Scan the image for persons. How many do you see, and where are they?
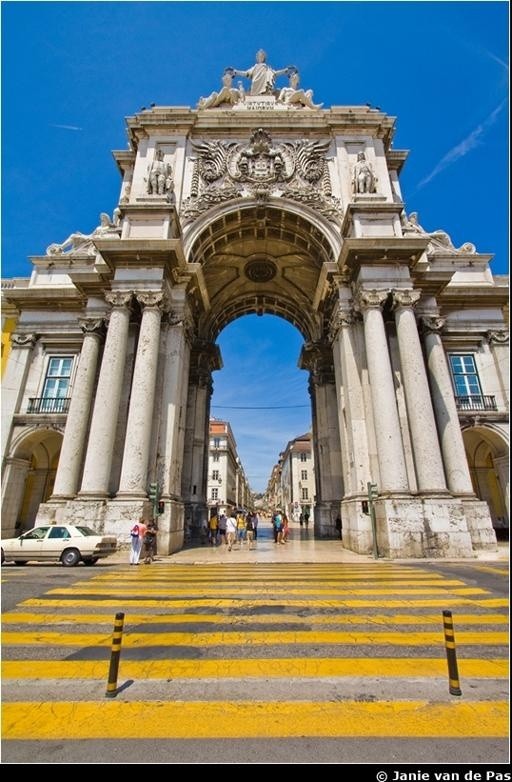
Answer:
[299,512,309,525]
[130,518,158,565]
[196,49,325,111]
[408,212,452,247]
[147,150,172,195]
[201,511,258,551]
[351,151,378,193]
[271,511,288,545]
[336,515,341,541]
[52,212,110,255]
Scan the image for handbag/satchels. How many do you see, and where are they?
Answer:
[131,525,139,536]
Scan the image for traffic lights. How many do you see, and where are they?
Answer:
[146,481,157,501]
[157,501,164,514]
[362,500,369,514]
[370,482,377,501]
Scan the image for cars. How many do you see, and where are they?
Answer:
[0,524,119,568]
